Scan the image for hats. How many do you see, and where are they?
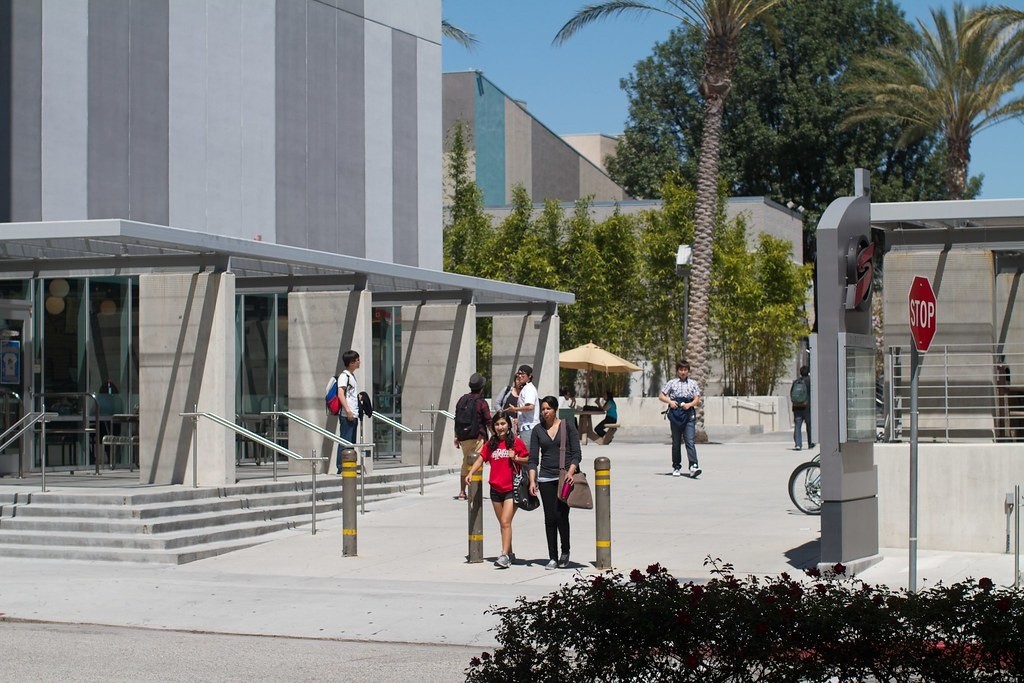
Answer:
[469,373,486,390]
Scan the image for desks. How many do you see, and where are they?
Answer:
[109,413,139,467]
[575,411,605,445]
[236,414,283,464]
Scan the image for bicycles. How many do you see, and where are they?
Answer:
[787,453,824,515]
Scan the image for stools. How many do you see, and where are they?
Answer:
[265,430,288,464]
[102,435,139,471]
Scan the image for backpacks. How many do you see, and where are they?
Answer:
[791,378,808,404]
[455,392,483,437]
[325,372,357,414]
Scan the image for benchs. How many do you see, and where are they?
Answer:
[604,424,620,445]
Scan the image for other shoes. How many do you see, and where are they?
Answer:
[795,446,801,450]
[808,443,816,449]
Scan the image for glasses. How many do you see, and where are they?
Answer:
[516,372,527,376]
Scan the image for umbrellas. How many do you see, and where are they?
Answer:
[559,340,644,405]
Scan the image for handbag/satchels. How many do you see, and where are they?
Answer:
[558,470,593,509]
[666,404,687,427]
[513,470,541,511]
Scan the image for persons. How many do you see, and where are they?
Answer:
[465,412,529,569]
[594,393,618,438]
[659,360,702,478]
[454,375,495,500]
[336,350,363,477]
[560,391,578,429]
[791,366,816,449]
[495,365,540,451]
[529,396,582,569]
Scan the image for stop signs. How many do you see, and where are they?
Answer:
[908,275,938,353]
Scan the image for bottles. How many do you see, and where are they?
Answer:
[561,478,573,501]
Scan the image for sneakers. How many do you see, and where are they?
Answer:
[689,463,702,479]
[494,555,511,567]
[498,554,517,562]
[544,559,558,570]
[559,551,570,568]
[673,468,680,476]
